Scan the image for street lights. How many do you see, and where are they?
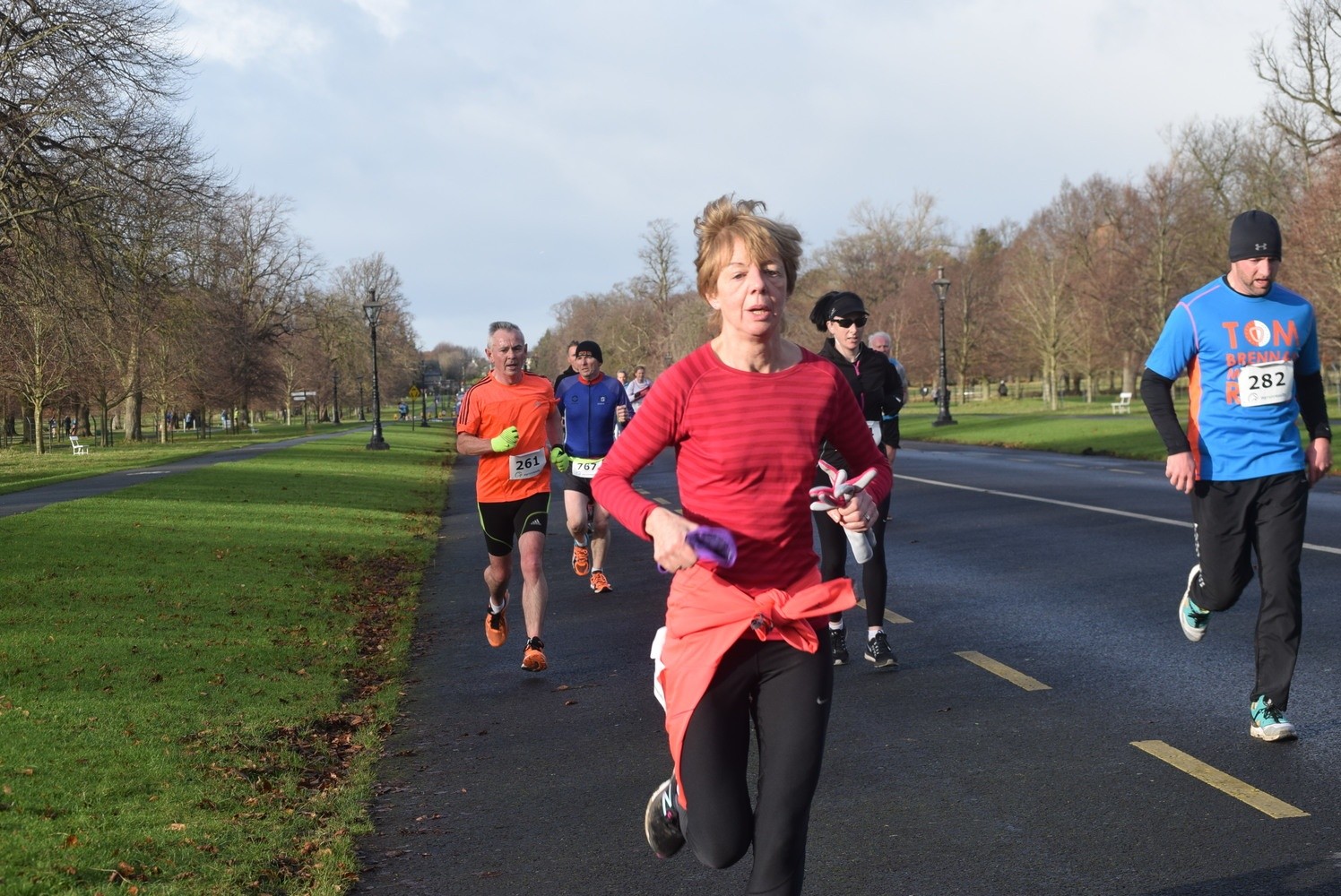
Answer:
[930,266,959,427]
[360,287,391,451]
[417,359,431,427]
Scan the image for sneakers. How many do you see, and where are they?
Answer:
[520,636,547,671]
[485,589,509,648]
[590,570,613,593]
[829,619,848,664]
[864,630,899,672]
[644,764,685,860]
[1178,564,1209,642]
[1250,696,1298,741]
[572,533,590,577]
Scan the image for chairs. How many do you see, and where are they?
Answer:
[1111,393,1132,414]
[69,436,89,455]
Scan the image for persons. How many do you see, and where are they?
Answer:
[1140,210,1332,742]
[234,408,238,426]
[398,401,409,421]
[50,415,78,438]
[455,321,652,673]
[186,413,193,430]
[590,196,893,896]
[998,380,1008,396]
[931,386,950,406]
[221,410,228,430]
[812,292,910,669]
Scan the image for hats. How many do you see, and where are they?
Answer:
[575,340,603,363]
[1229,210,1282,262]
[825,291,870,320]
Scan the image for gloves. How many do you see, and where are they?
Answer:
[807,460,878,565]
[490,425,519,453]
[550,444,569,473]
[656,525,738,576]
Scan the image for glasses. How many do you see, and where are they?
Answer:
[831,317,868,328]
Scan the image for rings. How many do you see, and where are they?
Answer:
[863,514,870,522]
